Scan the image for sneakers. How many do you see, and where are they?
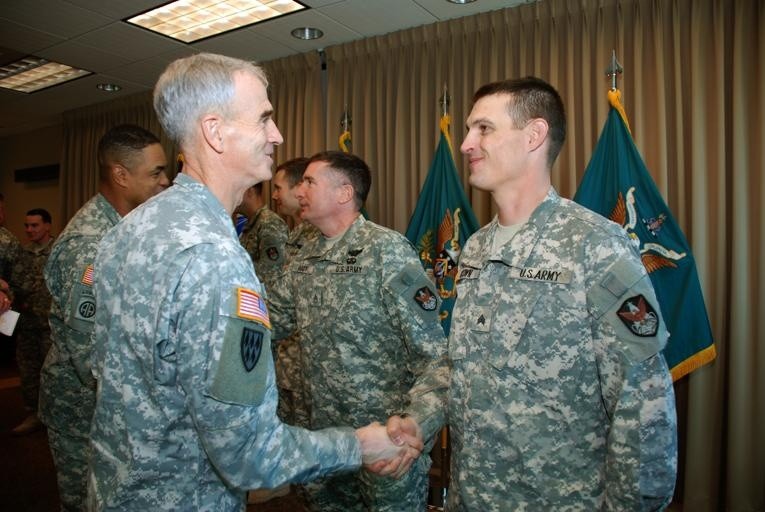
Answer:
[12,415,39,434]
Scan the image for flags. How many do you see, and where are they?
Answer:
[338,128,371,222]
[571,85,716,386]
[405,113,482,337]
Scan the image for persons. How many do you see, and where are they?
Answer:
[36,122,170,512]
[85,49,423,510]
[1,155,316,511]
[367,75,679,512]
[265,150,447,512]
